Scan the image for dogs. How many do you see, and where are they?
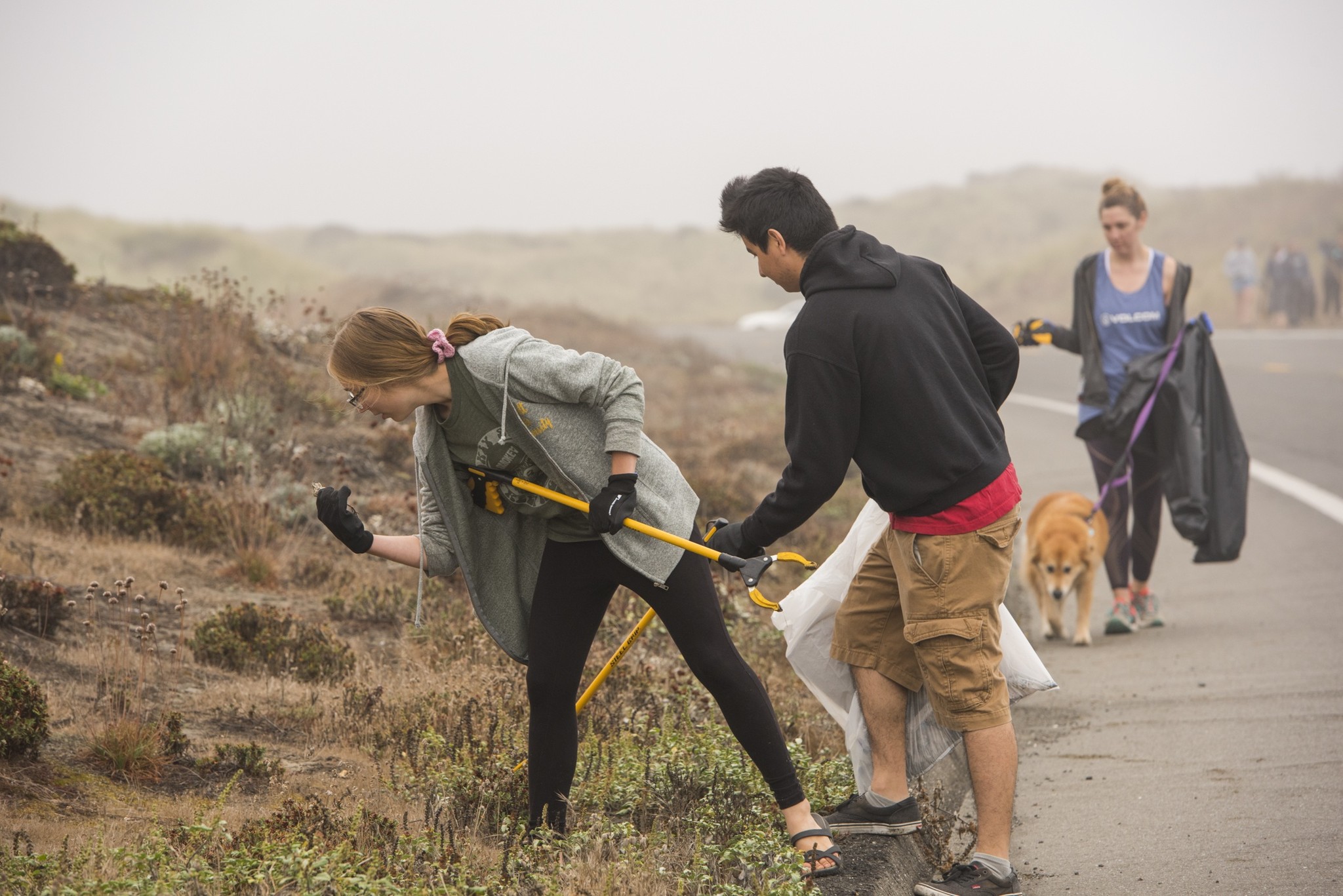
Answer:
[1018,489,1113,648]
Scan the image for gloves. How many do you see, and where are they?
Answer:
[316,485,374,554]
[589,473,638,536]
[1012,317,1059,347]
[705,523,747,562]
[1188,312,1214,336]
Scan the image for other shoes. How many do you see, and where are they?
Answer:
[1104,598,1136,634]
[1128,582,1164,628]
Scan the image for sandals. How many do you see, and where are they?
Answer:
[791,812,844,878]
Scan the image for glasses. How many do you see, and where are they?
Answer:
[347,388,365,410]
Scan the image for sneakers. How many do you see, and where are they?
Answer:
[914,860,1022,896]
[823,792,923,835]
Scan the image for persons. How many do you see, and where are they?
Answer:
[701,170,1028,896]
[1012,178,1211,632]
[312,308,846,879]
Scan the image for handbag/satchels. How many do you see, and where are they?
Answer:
[1163,327,1251,566]
[771,498,1060,798]
